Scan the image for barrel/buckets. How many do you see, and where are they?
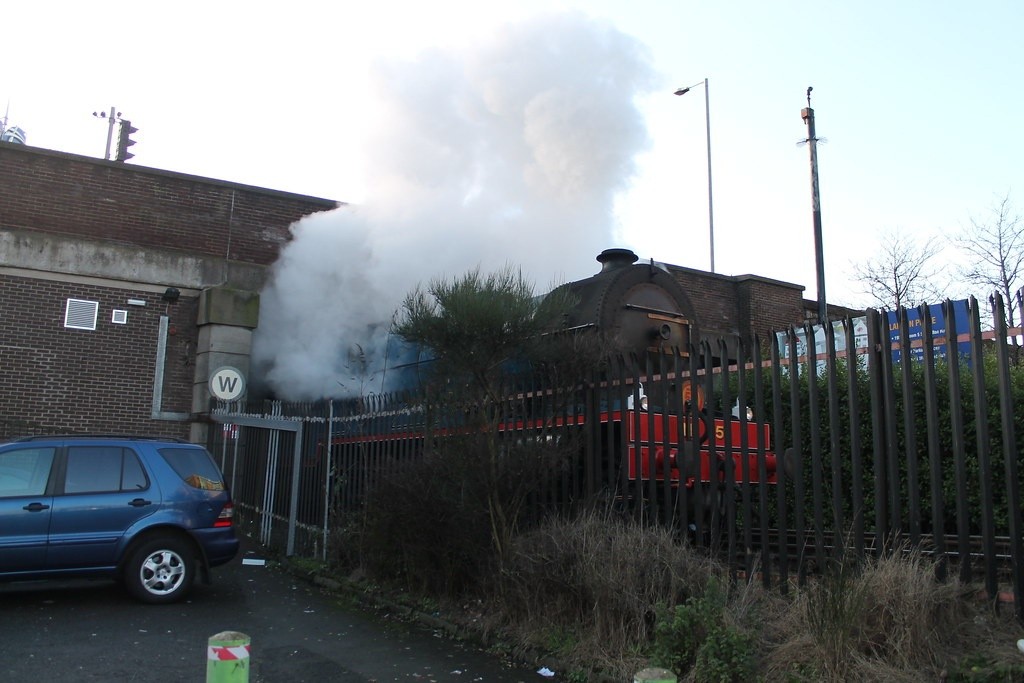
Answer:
[634,669,678,683]
[206,631,250,683]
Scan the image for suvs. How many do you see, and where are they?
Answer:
[1,435,241,603]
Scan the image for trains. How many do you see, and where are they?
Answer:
[276,249,778,523]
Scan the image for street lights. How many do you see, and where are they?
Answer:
[673,79,715,271]
